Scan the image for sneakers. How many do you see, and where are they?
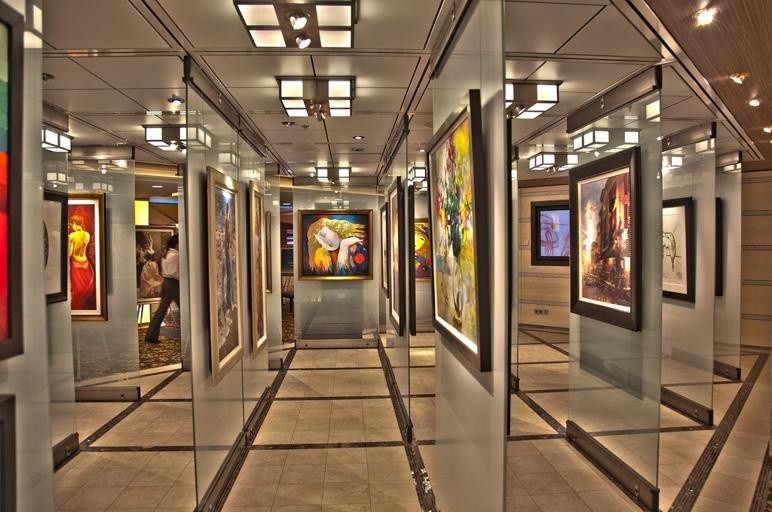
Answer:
[145,338,161,343]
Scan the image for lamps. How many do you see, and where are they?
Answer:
[233,0,357,122]
[693,133,742,173]
[141,122,214,155]
[502,79,564,121]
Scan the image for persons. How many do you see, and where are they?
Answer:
[139,250,165,300]
[68,214,96,310]
[145,233,179,344]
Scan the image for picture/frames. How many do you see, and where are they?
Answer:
[0,1,107,366]
[137,223,180,304]
